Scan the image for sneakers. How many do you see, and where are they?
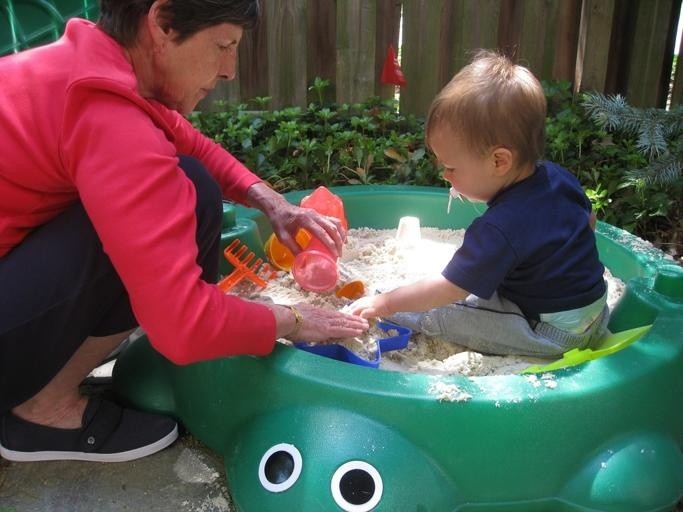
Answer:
[0,395,180,464]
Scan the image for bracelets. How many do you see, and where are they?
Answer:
[280,303,303,340]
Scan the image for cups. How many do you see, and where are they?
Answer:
[265,227,311,272]
[397,216,421,242]
[290,228,338,293]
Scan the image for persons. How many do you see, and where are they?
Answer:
[0,0,370,463]
[346,43,610,358]
